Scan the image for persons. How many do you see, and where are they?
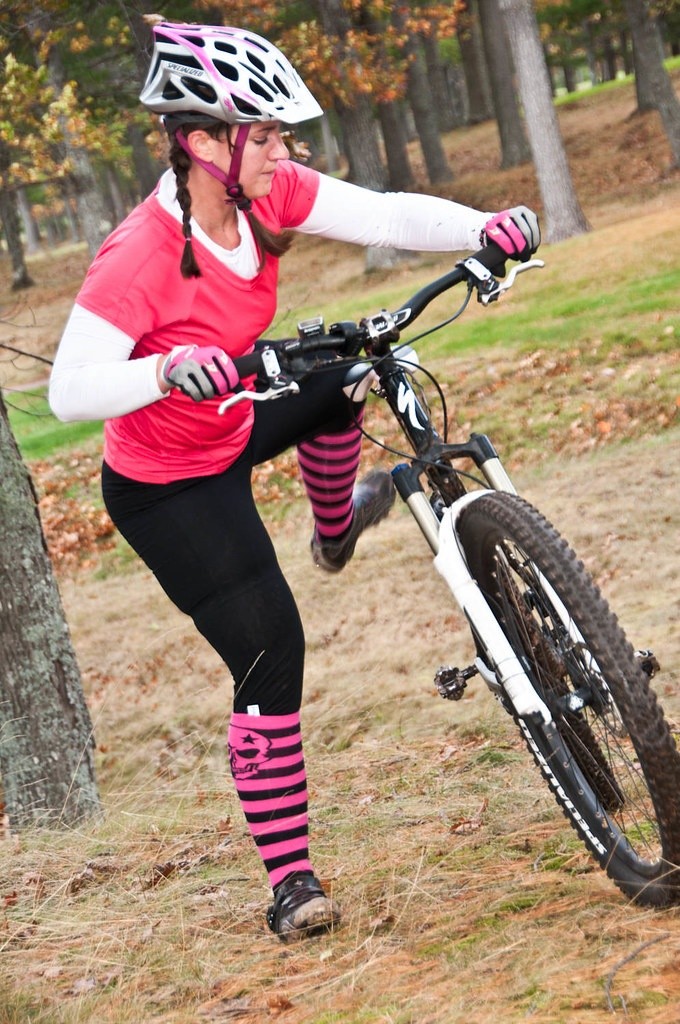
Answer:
[50,20,545,950]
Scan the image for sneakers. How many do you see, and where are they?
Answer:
[265,875,341,945]
[310,469,397,575]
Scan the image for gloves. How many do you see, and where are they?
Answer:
[479,205,542,264]
[160,345,247,403]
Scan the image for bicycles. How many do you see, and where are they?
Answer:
[173,238,680,911]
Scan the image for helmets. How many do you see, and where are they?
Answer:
[138,22,325,127]
